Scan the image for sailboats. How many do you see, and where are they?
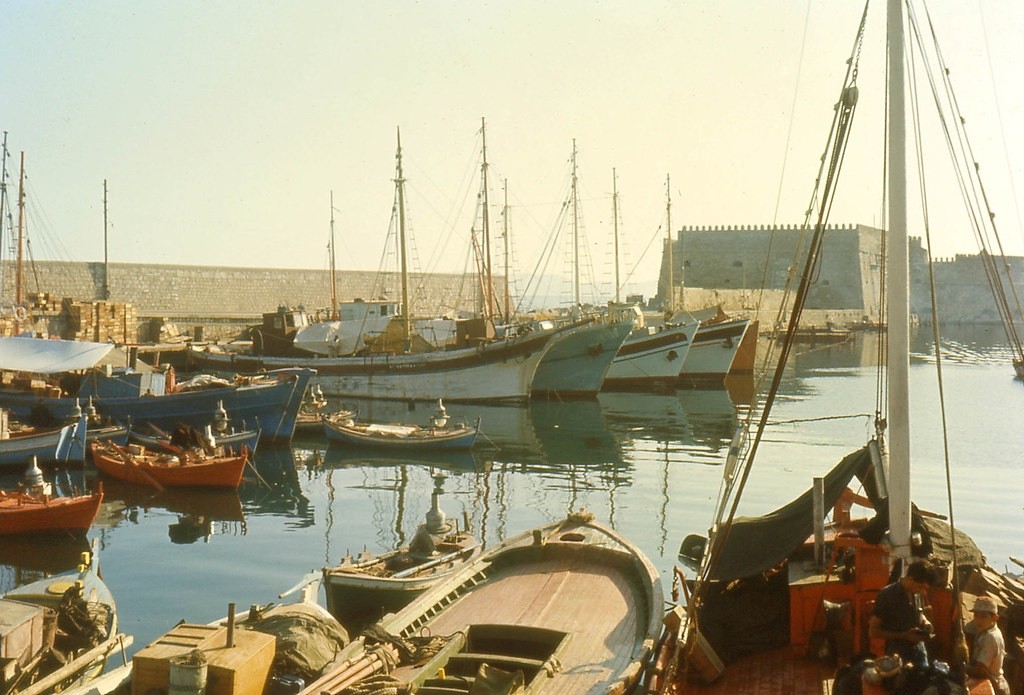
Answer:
[183,117,761,406]
[638,0,1023,694]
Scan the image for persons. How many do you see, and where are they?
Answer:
[965,596,1011,695]
[869,560,937,666]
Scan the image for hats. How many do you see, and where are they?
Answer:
[968,596,998,614]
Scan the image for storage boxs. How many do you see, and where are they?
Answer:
[22,379,65,399]
[1,292,139,345]
[660,605,725,685]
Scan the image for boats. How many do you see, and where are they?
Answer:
[0,536,118,695]
[58,572,350,695]
[320,398,482,453]
[295,408,357,434]
[0,396,132,471]
[321,489,482,620]
[294,505,664,694]
[0,534,103,580]
[91,437,248,489]
[320,440,486,475]
[0,454,104,538]
[90,473,247,545]
[0,334,320,452]
[777,320,851,341]
